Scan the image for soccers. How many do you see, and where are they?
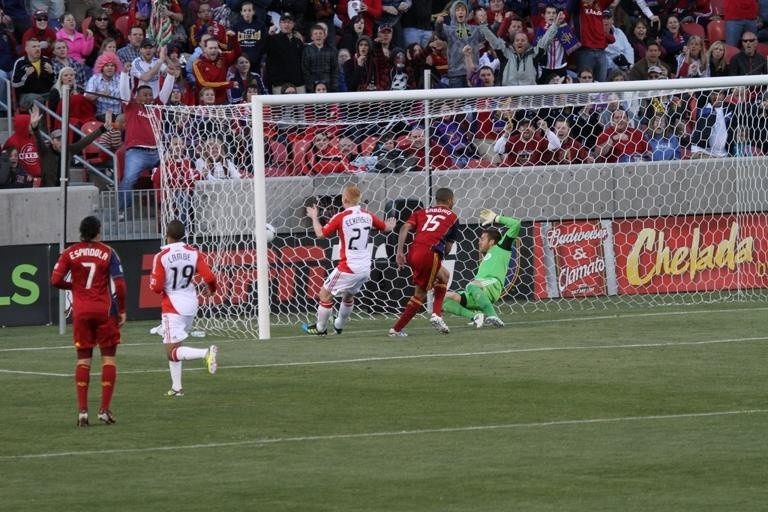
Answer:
[252,223,277,243]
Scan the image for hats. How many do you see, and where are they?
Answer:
[377,22,394,34]
[602,9,612,19]
[141,37,157,48]
[279,12,296,21]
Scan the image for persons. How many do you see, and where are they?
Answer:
[678,0,715,26]
[478,7,567,86]
[190,4,228,51]
[185,32,235,89]
[29,104,113,187]
[639,0,663,43]
[390,49,409,90]
[628,19,667,62]
[382,0,412,51]
[198,86,233,138]
[10,38,56,109]
[431,105,482,170]
[87,8,125,60]
[493,115,561,165]
[399,129,454,168]
[49,39,86,92]
[194,138,241,183]
[149,220,219,398]
[592,110,653,162]
[555,117,588,164]
[301,25,342,94]
[160,89,193,150]
[0,10,18,111]
[441,207,521,329]
[50,215,126,428]
[547,72,573,91]
[302,184,396,337]
[396,1,449,50]
[246,13,304,90]
[609,70,628,81]
[83,51,123,121]
[334,0,383,41]
[116,26,146,73]
[374,23,395,91]
[307,81,341,126]
[137,0,183,52]
[435,0,503,87]
[488,0,507,27]
[373,133,410,173]
[407,40,447,88]
[339,135,373,172]
[335,47,355,93]
[648,65,666,80]
[56,12,94,67]
[629,44,671,80]
[599,8,635,72]
[1,144,35,189]
[707,38,729,77]
[497,12,528,41]
[462,44,496,87]
[47,66,82,130]
[729,31,768,75]
[578,0,620,82]
[734,125,756,158]
[676,35,711,79]
[128,38,175,104]
[473,7,488,26]
[169,57,184,90]
[117,59,177,221]
[344,35,379,91]
[669,108,694,154]
[228,3,277,60]
[318,22,328,40]
[241,85,261,104]
[533,4,581,85]
[722,0,759,47]
[576,66,594,82]
[432,33,450,56]
[21,9,56,59]
[309,132,348,174]
[389,187,459,337]
[96,37,122,70]
[226,53,267,104]
[274,82,306,151]
[641,110,680,159]
[152,137,200,224]
[661,17,692,56]
[694,89,738,160]
[337,14,368,58]
[193,29,242,106]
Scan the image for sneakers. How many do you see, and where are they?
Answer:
[96,407,117,425]
[387,327,408,338]
[159,387,186,398]
[472,312,486,330]
[204,344,218,374]
[484,315,505,330]
[76,409,90,428]
[328,315,343,336]
[428,313,451,336]
[304,324,328,338]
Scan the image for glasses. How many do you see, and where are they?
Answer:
[741,38,758,43]
[94,17,110,22]
[197,9,212,14]
[280,20,293,25]
[34,17,48,22]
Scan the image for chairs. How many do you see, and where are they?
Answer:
[681,24,704,37]
[269,141,288,161]
[82,17,92,34]
[12,87,27,115]
[726,44,740,64]
[756,43,768,57]
[397,135,412,148]
[46,101,54,133]
[81,122,108,169]
[239,170,253,178]
[466,160,494,169]
[115,145,152,189]
[707,20,725,44]
[292,140,311,166]
[361,137,379,156]
[116,16,137,40]
[265,167,289,177]
